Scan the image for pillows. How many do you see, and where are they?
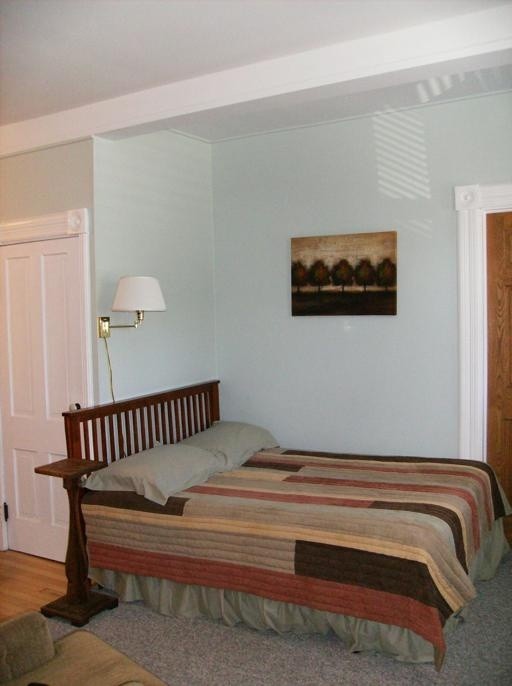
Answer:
[180,420,282,469]
[82,444,226,507]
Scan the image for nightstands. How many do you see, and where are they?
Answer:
[34,454,118,627]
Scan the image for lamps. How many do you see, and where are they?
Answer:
[97,276,166,339]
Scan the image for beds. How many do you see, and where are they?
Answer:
[59,379,512,669]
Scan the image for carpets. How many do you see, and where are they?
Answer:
[39,554,512,685]
[0,613,168,686]
